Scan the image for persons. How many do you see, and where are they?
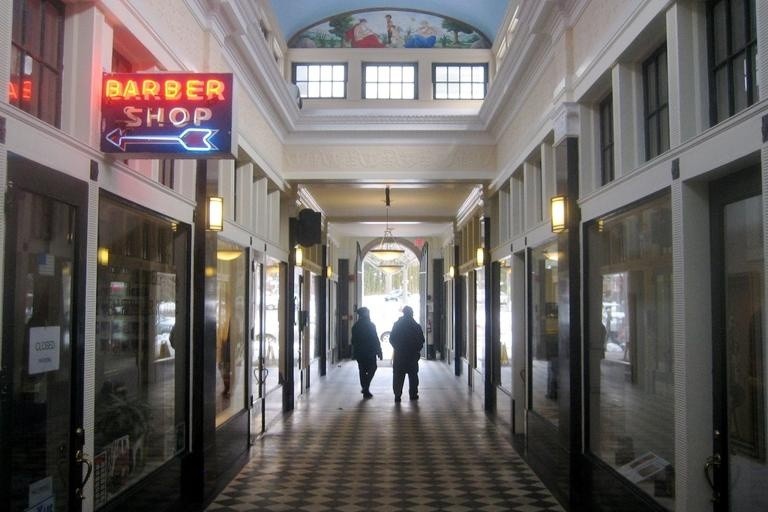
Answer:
[385,15,396,45]
[414,20,437,38]
[352,17,379,43]
[388,305,425,402]
[350,305,383,399]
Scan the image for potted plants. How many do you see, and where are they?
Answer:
[96,388,155,477]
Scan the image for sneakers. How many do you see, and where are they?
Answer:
[410,394,419,399]
[395,397,401,402]
[361,389,373,399]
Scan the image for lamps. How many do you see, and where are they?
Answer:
[207,195,223,233]
[379,265,404,276]
[549,195,566,233]
[295,248,303,267]
[368,187,404,263]
[218,249,242,261]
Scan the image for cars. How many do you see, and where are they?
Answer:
[363,290,420,343]
